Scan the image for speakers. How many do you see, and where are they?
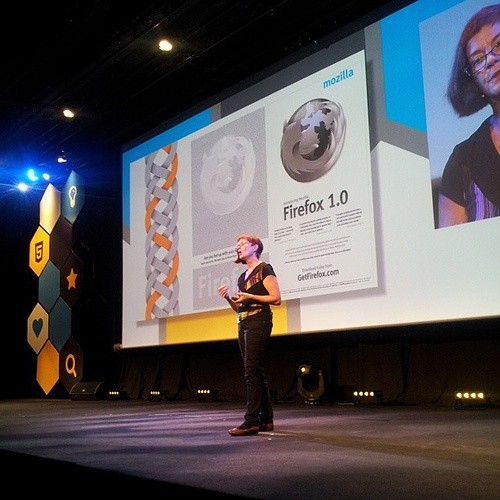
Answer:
[69,382,106,402]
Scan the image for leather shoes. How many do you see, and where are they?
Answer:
[259,422,273,430]
[229,426,259,435]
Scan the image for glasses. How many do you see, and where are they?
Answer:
[464,41,500,76]
[235,241,252,249]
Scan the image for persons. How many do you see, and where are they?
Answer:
[217,234,282,437]
[439,3,500,227]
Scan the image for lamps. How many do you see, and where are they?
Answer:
[149,389,161,402]
[353,390,385,408]
[196,388,218,402]
[106,390,121,400]
[453,390,489,411]
[298,364,312,378]
